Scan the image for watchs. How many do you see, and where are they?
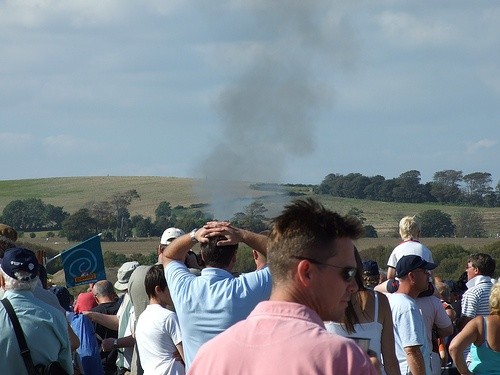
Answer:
[113,339,119,349]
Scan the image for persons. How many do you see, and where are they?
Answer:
[1,196,500,375]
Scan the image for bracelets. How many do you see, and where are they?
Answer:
[190,227,200,245]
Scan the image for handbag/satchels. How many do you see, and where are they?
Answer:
[35,361,69,375]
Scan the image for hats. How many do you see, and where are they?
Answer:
[0,248,39,281]
[361,260,380,276]
[114,261,140,290]
[396,254,437,278]
[48,286,70,306]
[161,228,185,245]
[0,224,17,242]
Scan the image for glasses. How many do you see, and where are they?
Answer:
[465,265,472,269]
[289,256,357,283]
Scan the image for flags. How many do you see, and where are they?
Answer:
[60,234,107,290]
